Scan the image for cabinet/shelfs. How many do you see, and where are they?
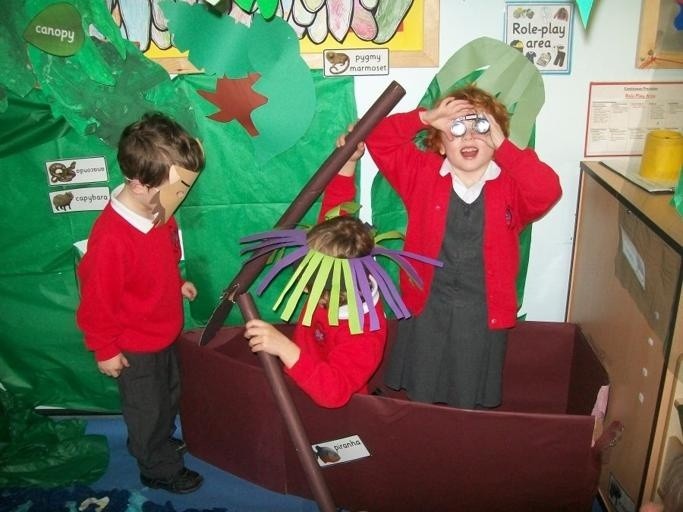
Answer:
[566,161,682,511]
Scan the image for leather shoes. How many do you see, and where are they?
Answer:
[127,438,203,493]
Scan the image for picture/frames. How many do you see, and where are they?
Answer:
[502,1,576,74]
[633,1,682,70]
[89,1,440,71]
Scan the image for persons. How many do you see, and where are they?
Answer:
[244,124,388,411]
[76,113,204,494]
[363,85,562,408]
